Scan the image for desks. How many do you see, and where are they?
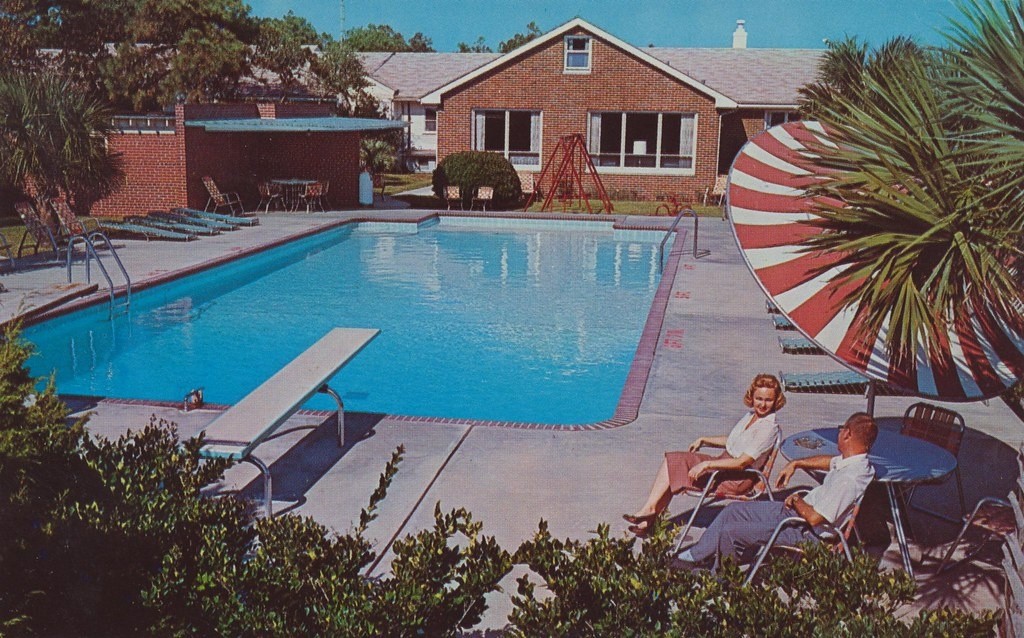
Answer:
[778,426,959,593]
[271,179,318,212]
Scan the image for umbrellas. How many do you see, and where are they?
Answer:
[726,120,1024,403]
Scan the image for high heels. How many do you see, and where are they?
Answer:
[623,514,656,527]
[629,526,655,537]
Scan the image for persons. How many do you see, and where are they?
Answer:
[623,372,786,536]
[673,411,878,581]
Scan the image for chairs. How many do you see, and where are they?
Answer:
[255,182,288,214]
[443,185,464,210]
[739,462,875,586]
[201,175,245,214]
[703,177,727,207]
[14,201,78,260]
[373,175,386,201]
[936,469,1024,612]
[673,426,784,556]
[49,196,108,249]
[469,185,495,212]
[889,402,967,544]
[0,233,16,269]
[295,182,325,214]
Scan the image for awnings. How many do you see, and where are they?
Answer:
[183,117,408,131]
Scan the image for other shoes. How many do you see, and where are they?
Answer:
[670,555,695,569]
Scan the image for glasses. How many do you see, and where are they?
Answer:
[838,425,848,431]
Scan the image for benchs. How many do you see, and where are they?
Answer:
[96,206,260,242]
[766,299,870,400]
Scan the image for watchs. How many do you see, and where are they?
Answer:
[790,495,800,507]
